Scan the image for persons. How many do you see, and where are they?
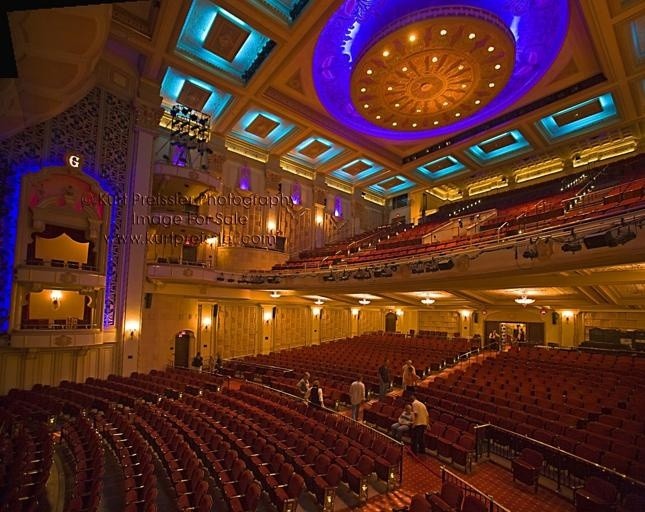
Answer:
[401,359,416,387]
[214,358,224,375]
[378,358,394,399]
[348,373,367,422]
[408,396,432,452]
[403,366,417,395]
[191,351,205,373]
[488,324,526,353]
[389,405,415,444]
[303,379,326,410]
[295,370,311,399]
